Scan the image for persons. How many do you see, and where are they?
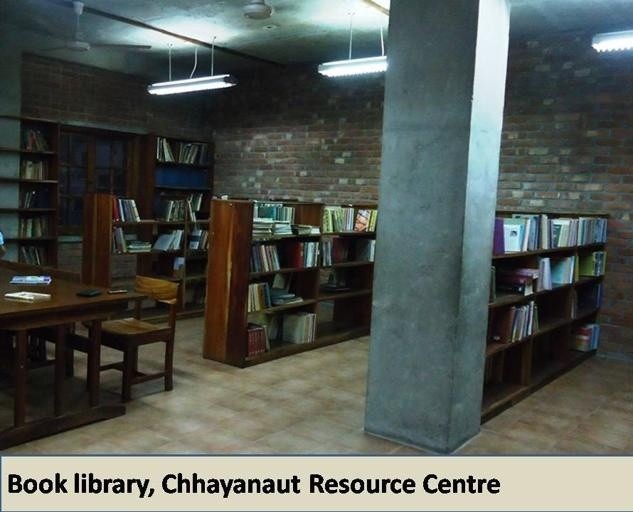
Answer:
[0,231,8,255]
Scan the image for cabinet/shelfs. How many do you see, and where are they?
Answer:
[82,134,214,329]
[1,113,60,269]
[204,197,378,369]
[480,212,608,422]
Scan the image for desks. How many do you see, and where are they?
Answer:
[0,257,124,453]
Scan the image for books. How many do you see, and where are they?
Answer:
[247,200,321,357]
[113,192,211,254]
[491,214,608,352]
[173,257,186,279]
[321,205,378,292]
[156,136,208,164]
[20,124,50,266]
[4,291,51,300]
[8,276,52,285]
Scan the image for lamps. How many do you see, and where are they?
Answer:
[316,0,387,79]
[144,38,237,98]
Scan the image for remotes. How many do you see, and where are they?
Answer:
[108,289,129,294]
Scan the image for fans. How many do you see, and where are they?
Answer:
[23,4,101,55]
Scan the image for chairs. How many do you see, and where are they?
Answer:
[84,274,178,403]
